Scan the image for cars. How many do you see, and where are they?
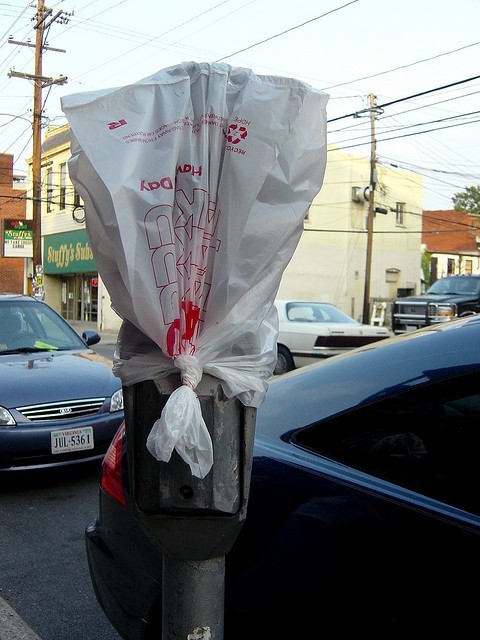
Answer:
[87,315,480,638]
[273,301,390,374]
[0,293,128,478]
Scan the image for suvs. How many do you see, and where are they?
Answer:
[393,274,480,334]
